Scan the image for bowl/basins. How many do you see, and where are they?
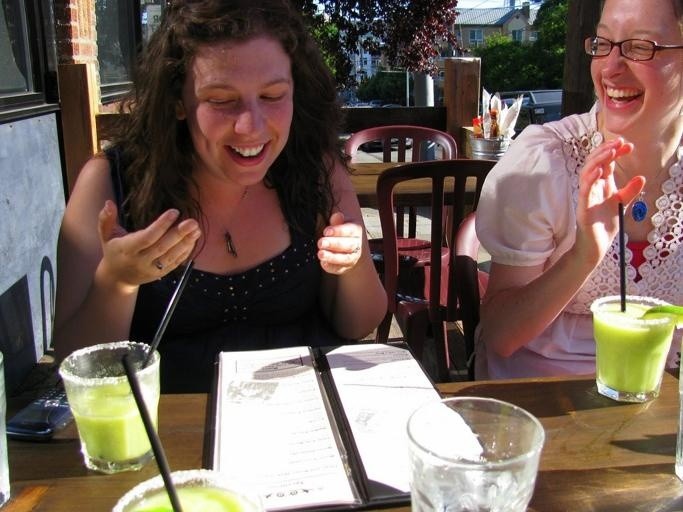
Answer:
[469,138,510,157]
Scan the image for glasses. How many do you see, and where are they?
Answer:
[583,33,683,60]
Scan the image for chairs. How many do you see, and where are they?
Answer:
[345,125,499,382]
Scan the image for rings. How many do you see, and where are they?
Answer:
[154,259,163,270]
[354,244,360,253]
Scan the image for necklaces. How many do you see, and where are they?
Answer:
[202,183,248,257]
[615,158,670,223]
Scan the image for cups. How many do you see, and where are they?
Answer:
[405,395,547,512]
[57,340,163,477]
[588,294,678,405]
[111,468,265,512]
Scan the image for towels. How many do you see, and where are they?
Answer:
[462,87,523,139]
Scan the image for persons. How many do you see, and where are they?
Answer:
[53,2,389,394]
[473,1,683,380]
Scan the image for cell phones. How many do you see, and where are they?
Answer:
[4,383,73,441]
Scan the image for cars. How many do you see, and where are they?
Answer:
[492,90,563,134]
[352,100,412,152]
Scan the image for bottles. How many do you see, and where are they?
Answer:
[489,108,498,137]
[472,118,485,138]
